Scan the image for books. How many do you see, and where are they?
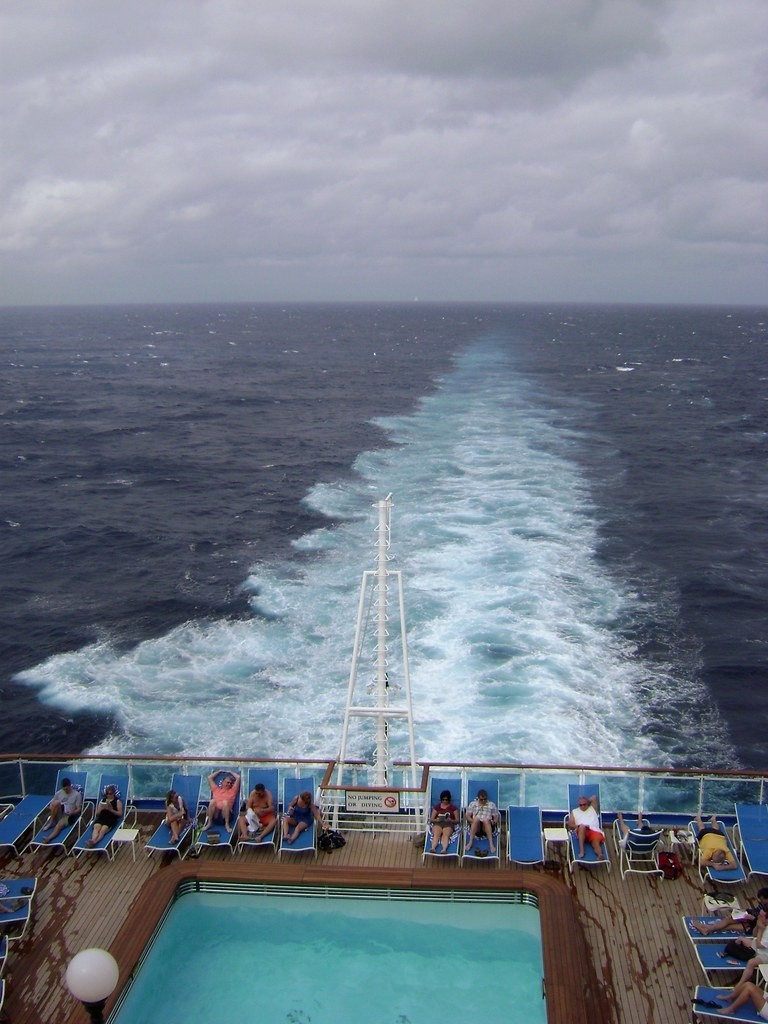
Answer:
[100,802,108,809]
[437,811,451,819]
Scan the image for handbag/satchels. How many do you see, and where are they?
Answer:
[317,829,346,851]
[414,833,425,847]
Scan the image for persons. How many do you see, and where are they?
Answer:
[238,783,276,843]
[568,795,606,860]
[690,887,768,1021]
[617,812,650,849]
[201,770,241,832]
[464,790,499,853]
[694,812,738,870]
[42,778,82,843]
[431,789,460,854]
[84,784,122,848]
[282,791,330,845]
[165,790,188,845]
[0,904,15,913]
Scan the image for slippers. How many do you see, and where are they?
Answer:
[704,1000,722,1009]
[691,998,708,1006]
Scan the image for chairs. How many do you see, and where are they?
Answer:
[0,768,768,1024]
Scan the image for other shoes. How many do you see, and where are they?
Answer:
[475,848,481,856]
[480,849,488,857]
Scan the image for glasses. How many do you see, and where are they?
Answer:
[255,791,263,794]
[442,799,448,801]
[225,780,232,784]
[63,787,69,790]
[579,802,587,806]
[106,792,114,795]
[479,798,486,801]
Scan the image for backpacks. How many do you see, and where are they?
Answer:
[658,852,682,880]
